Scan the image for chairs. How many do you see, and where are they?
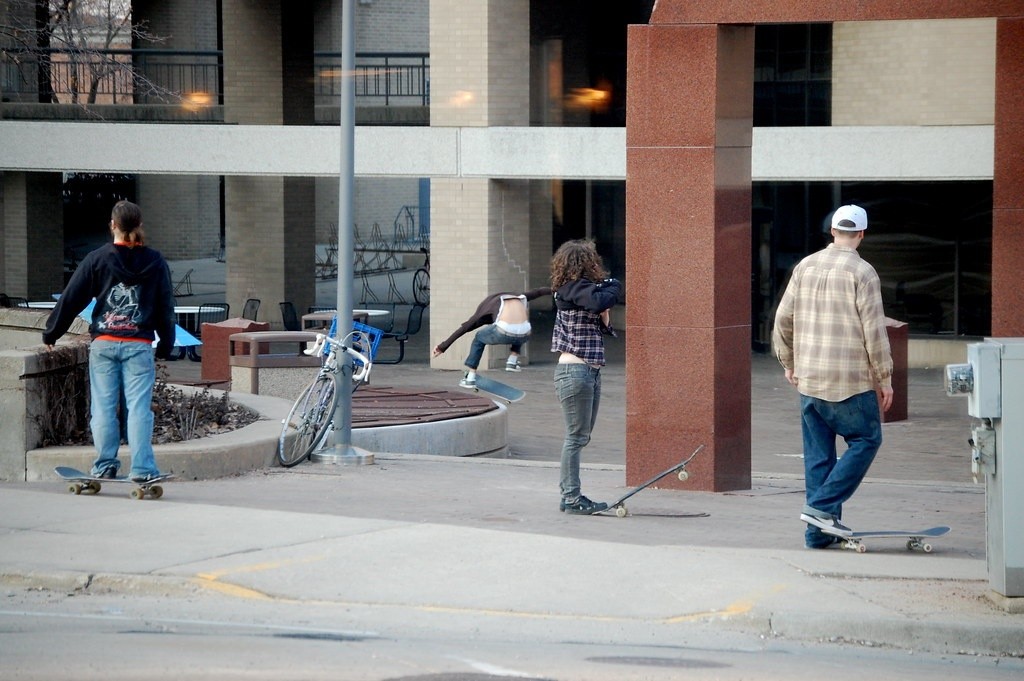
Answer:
[279,301,427,364]
[0,293,29,310]
[191,298,260,362]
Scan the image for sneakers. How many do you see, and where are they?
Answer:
[564,495,608,515]
[799,511,854,546]
[132,471,159,482]
[457,373,477,389]
[559,497,566,511]
[505,360,522,374]
[90,464,117,479]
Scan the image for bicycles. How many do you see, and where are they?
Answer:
[277,315,386,469]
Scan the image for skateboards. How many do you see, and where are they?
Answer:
[590,444,704,518]
[465,370,526,405]
[820,526,952,553]
[54,466,176,499]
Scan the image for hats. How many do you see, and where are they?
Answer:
[830,203,868,231]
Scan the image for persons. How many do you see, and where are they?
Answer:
[43,199,177,482]
[432,287,553,389]
[772,204,893,549]
[549,239,622,514]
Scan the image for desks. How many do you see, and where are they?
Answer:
[173,306,226,361]
[312,309,391,362]
[18,302,57,309]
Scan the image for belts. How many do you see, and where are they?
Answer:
[496,326,531,337]
